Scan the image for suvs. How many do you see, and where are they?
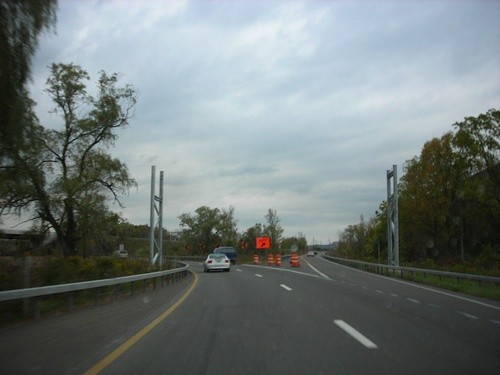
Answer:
[114,250,128,259]
[212,247,239,264]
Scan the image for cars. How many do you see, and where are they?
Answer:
[308,250,318,257]
[204,254,231,272]
